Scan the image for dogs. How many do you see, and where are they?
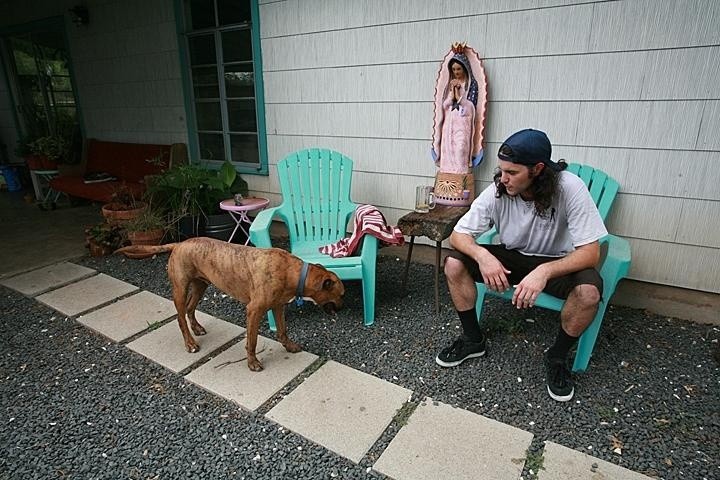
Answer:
[108,234,348,371]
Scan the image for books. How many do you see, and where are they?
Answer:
[82,169,118,187]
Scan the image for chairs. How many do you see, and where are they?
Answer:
[248,147,382,332]
[474,163,631,374]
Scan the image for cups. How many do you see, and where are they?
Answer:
[414,186,437,215]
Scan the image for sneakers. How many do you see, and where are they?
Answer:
[543,350,576,403]
[436,334,486,368]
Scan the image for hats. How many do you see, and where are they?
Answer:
[498,128,564,172]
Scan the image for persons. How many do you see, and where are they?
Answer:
[434,126,610,404]
[438,54,480,176]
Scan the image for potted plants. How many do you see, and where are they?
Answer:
[13,128,72,170]
[82,154,249,257]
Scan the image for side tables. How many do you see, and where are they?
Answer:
[220,196,270,246]
[397,202,470,312]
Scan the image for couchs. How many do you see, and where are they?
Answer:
[42,138,187,211]
